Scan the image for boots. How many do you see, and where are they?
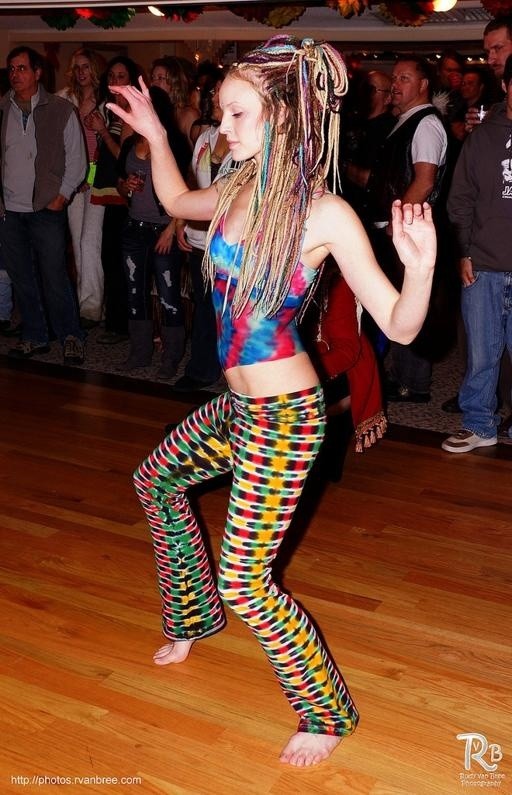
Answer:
[157,326,186,381]
[115,321,156,372]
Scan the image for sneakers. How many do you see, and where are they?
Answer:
[384,381,433,402]
[98,329,128,345]
[8,340,49,360]
[440,429,498,453]
[63,337,86,366]
[441,392,503,414]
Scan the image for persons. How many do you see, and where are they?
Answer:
[102,32,439,768]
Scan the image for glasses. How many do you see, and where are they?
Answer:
[362,83,392,96]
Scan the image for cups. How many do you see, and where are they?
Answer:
[478,105,488,122]
[133,170,146,192]
